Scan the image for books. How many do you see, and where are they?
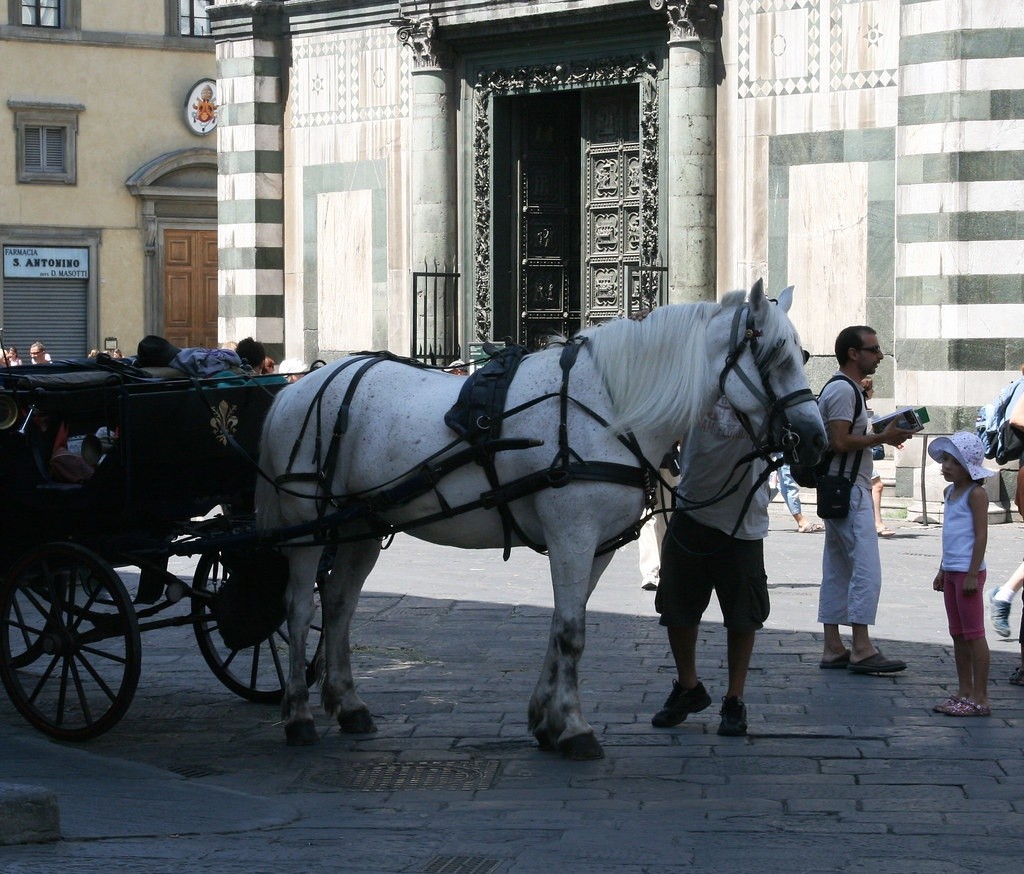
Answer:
[871,406,930,445]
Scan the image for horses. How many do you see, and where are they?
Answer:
[255,276,836,767]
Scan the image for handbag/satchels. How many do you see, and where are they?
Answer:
[816,475,850,519]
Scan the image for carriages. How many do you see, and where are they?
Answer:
[0,278,827,759]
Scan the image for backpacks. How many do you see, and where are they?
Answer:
[976,379,1024,460]
[790,375,868,488]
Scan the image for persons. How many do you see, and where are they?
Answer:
[860,376,904,536]
[0,339,53,369]
[976,363,1024,638]
[765,451,823,533]
[1009,557,1024,686]
[637,443,679,589]
[87,348,126,362]
[628,309,770,736]
[444,360,467,376]
[928,431,994,716]
[814,326,916,672]
[222,337,308,382]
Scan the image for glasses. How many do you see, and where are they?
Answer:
[857,345,880,354]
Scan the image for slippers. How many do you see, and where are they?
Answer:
[945,697,990,716]
[934,695,959,712]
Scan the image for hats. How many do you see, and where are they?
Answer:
[928,431,995,480]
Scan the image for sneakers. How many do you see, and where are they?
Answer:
[652,679,712,728]
[717,696,747,736]
[642,577,658,590]
[984,585,1011,637]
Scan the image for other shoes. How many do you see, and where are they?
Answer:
[799,522,823,534]
[848,645,907,673]
[878,526,895,536]
[819,649,851,669]
[1008,667,1024,685]
